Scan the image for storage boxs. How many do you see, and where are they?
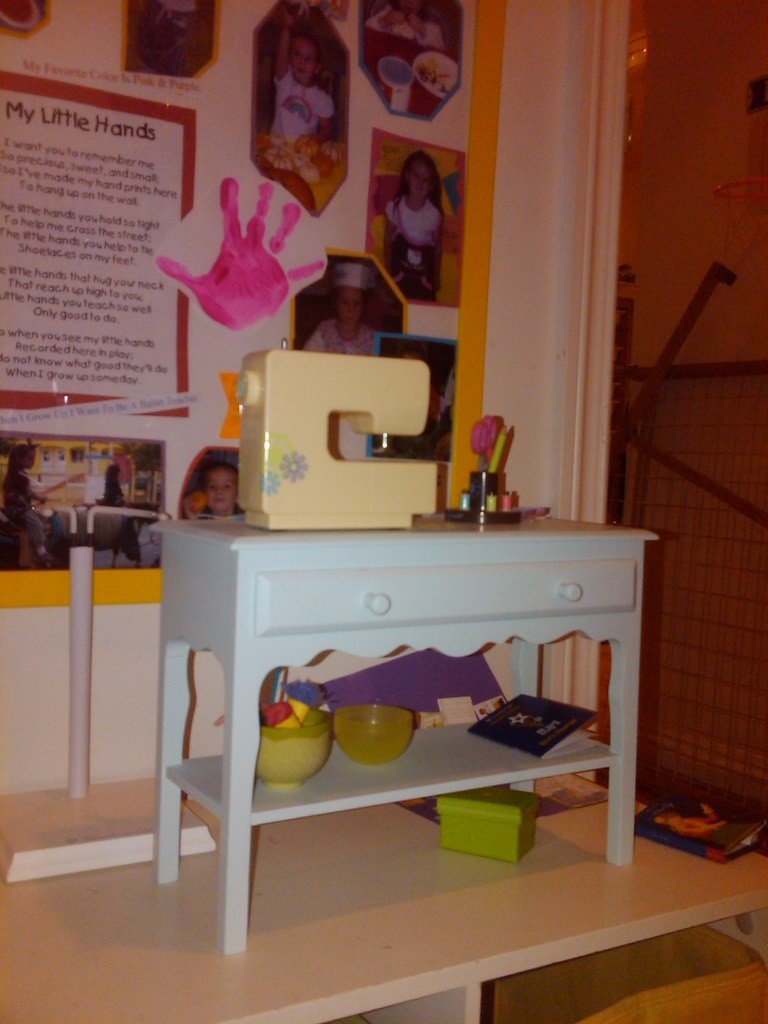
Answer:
[437,787,541,863]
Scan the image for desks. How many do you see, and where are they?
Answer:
[1,773,767,1024]
[155,515,656,958]
